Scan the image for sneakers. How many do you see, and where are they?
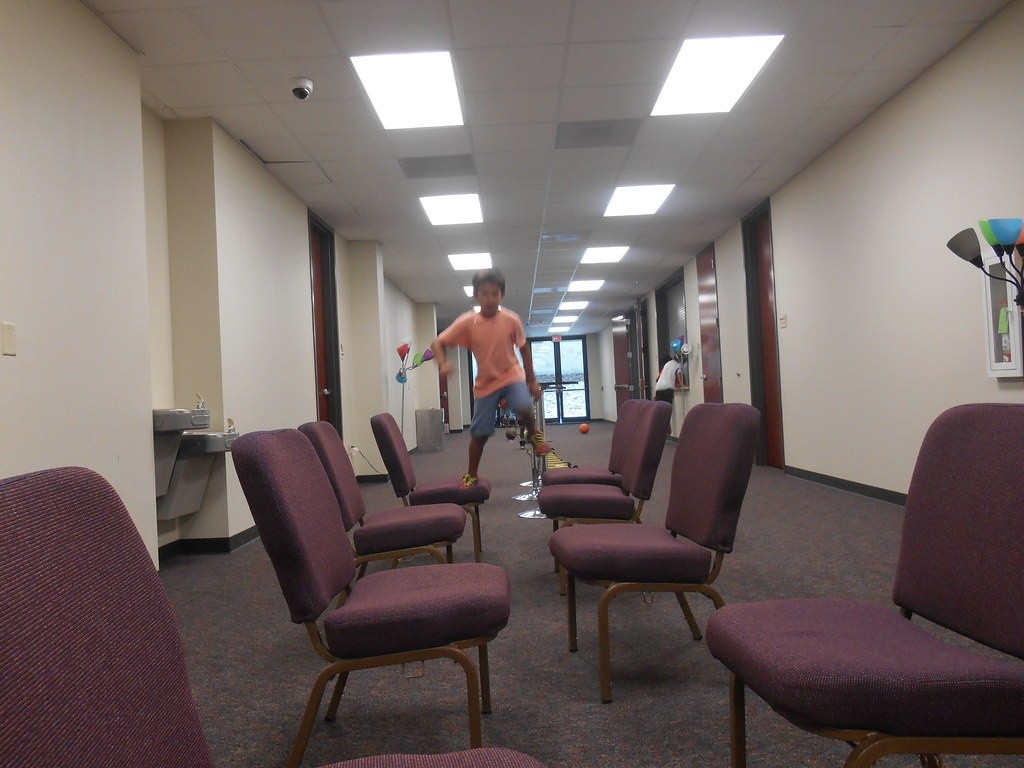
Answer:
[519,438,526,449]
[459,473,478,488]
[528,431,550,456]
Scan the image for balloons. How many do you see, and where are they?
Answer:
[670,339,691,355]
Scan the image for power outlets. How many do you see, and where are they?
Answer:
[347,443,357,457]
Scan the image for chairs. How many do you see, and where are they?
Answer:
[548,403,761,704]
[298,421,467,610]
[537,401,673,596]
[370,413,491,569]
[541,399,648,495]
[0,466,547,768]
[230,428,511,768]
[706,403,1024,768]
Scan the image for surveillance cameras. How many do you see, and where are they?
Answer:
[290,78,314,101]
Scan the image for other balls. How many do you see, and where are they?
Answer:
[579,423,589,433]
[524,431,529,440]
[506,428,516,439]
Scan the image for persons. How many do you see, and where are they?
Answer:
[432,265,553,488]
[654,351,685,436]
[515,412,530,449]
[498,396,511,428]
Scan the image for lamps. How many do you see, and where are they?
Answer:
[947,217,1024,376]
[395,336,436,438]
[670,335,692,422]
